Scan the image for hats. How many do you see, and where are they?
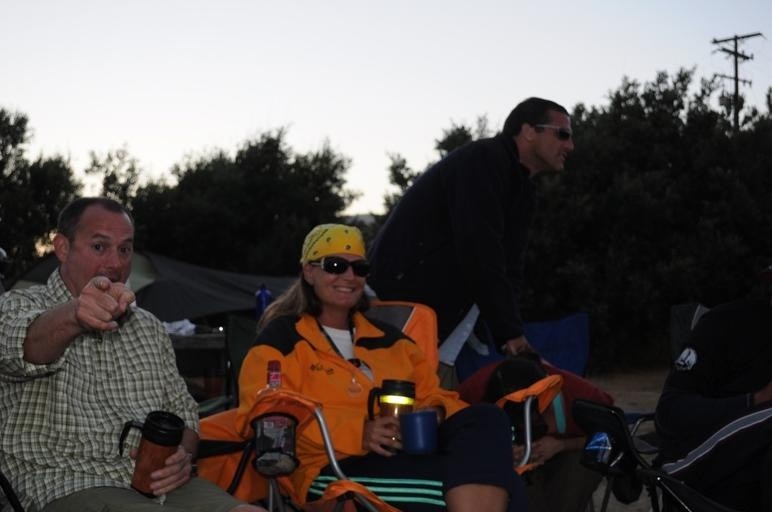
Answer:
[299,223,368,270]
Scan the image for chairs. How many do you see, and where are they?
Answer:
[494,372,564,470]
[196,391,384,510]
[572,399,733,511]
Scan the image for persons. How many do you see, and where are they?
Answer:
[362,92,575,385]
[238,221,515,511]
[1,198,263,511]
[651,293,772,509]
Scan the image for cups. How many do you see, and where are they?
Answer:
[367,380,416,452]
[398,412,438,457]
[118,410,185,499]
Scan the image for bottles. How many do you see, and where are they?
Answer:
[255,282,272,322]
[265,359,281,387]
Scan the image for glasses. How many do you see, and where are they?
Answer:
[307,256,369,277]
[538,124,575,141]
